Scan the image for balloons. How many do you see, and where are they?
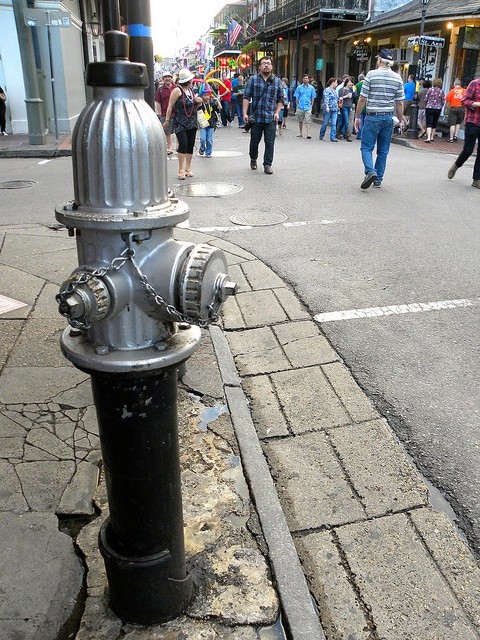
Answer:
[203,64,211,79]
[206,78,230,92]
[204,70,219,81]
[192,78,203,83]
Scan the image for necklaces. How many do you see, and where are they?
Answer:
[182,86,195,117]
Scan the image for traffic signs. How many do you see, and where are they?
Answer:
[419,35,445,48]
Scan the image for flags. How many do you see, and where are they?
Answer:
[243,20,249,37]
[204,42,215,63]
[195,41,202,54]
[229,21,241,47]
[225,25,232,50]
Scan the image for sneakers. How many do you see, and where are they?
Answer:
[417,130,426,138]
[336,134,342,140]
[471,180,480,188]
[229,120,234,128]
[448,163,458,179]
[345,135,352,141]
[222,124,226,127]
[251,159,257,169]
[374,182,382,187]
[239,125,245,128]
[283,124,286,128]
[361,172,377,188]
[264,165,272,174]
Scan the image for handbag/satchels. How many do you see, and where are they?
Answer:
[338,99,343,108]
[197,109,210,129]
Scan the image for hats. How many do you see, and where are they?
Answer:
[379,48,394,61]
[179,68,195,84]
[358,74,364,80]
[453,80,461,85]
[162,71,173,80]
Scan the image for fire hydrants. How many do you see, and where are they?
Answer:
[55,31,239,627]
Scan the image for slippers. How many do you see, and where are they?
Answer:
[206,155,211,157]
[199,148,204,155]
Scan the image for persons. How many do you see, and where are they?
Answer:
[311,75,323,118]
[155,71,179,156]
[0,88,8,137]
[154,75,163,91]
[218,72,233,127]
[233,76,247,127]
[336,74,354,141]
[352,73,366,140]
[162,68,203,180]
[337,78,356,142]
[293,74,317,139]
[418,75,432,139]
[281,77,290,128]
[446,80,467,143]
[290,74,300,113]
[276,101,284,136]
[229,72,240,120]
[197,92,223,158]
[403,74,416,132]
[354,49,406,189]
[242,56,284,174]
[424,79,446,143]
[175,74,180,84]
[448,78,480,190]
[319,78,339,142]
[419,77,425,90]
[244,77,252,114]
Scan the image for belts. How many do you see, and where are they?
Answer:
[367,112,393,116]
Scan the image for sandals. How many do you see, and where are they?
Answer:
[185,168,193,177]
[453,136,458,141]
[178,170,186,180]
[447,139,454,143]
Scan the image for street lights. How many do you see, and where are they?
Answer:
[407,0,429,139]
